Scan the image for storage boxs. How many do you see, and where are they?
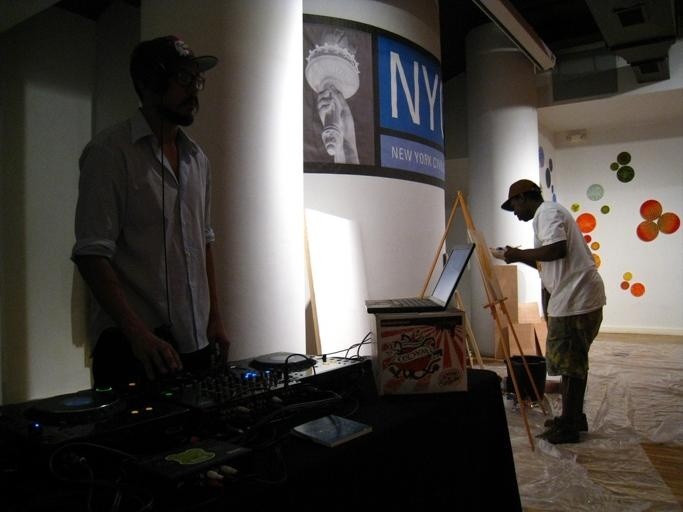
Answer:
[371,301,468,395]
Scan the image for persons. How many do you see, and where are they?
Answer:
[70,35,231,388]
[317,85,360,165]
[496,180,606,445]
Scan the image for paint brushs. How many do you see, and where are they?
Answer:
[489,247,507,251]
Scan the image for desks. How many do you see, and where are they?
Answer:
[2,363,516,511]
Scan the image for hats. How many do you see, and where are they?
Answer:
[501,179,541,210]
[131,35,219,72]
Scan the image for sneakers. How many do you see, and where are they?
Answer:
[536,414,589,444]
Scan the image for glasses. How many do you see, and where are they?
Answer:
[177,70,205,92]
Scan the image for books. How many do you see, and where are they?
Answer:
[290,413,373,448]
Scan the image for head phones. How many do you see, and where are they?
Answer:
[142,40,170,96]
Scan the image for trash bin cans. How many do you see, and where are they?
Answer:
[504,355,546,402]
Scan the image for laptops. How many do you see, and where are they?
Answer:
[363,242,476,314]
